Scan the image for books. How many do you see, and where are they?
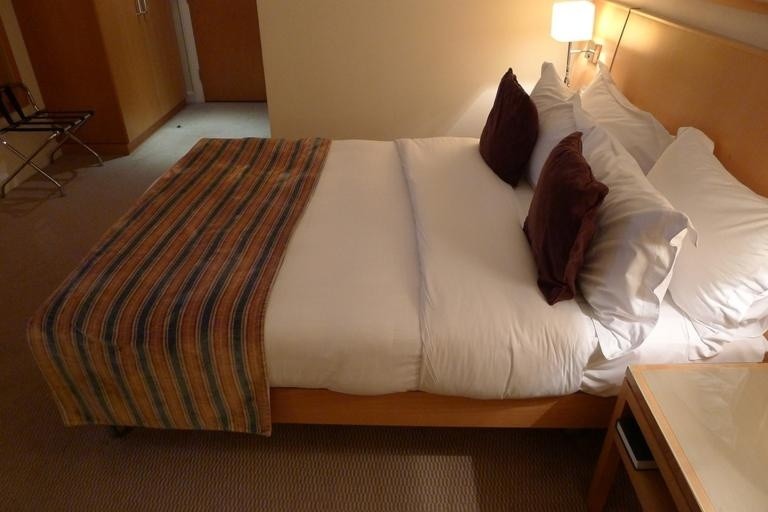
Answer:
[614,417,660,472]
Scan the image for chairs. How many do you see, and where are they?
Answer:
[1,82,102,198]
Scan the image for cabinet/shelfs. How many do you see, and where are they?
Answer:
[10,0,188,156]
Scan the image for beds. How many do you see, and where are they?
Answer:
[29,10,767,440]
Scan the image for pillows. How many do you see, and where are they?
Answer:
[529,61,596,193]
[521,130,609,308]
[643,128,768,361]
[579,61,676,175]
[480,66,543,193]
[575,122,700,361]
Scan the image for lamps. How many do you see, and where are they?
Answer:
[550,0,603,87]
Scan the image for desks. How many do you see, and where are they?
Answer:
[589,362,768,512]
